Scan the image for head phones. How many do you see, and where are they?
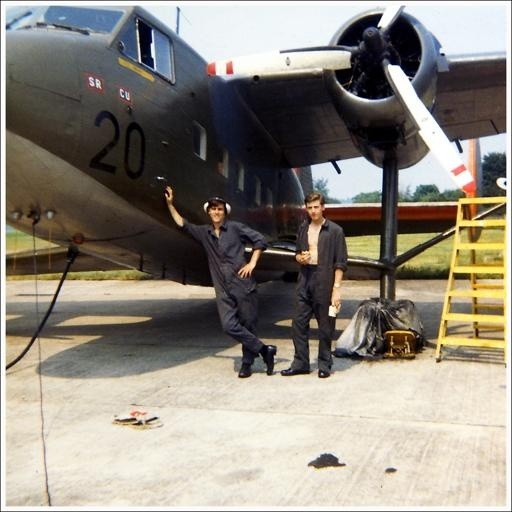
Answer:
[202,196,232,216]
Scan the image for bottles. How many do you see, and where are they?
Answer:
[328,302,337,318]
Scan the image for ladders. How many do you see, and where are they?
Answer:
[434,196,507,366]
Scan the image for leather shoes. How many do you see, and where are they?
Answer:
[318,366,331,378]
[281,367,310,375]
[239,363,251,377]
[262,345,277,375]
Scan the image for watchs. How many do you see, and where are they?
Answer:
[333,281,342,288]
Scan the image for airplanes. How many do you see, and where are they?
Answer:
[5,3,508,289]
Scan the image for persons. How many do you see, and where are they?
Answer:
[279,190,346,380]
[164,185,276,379]
